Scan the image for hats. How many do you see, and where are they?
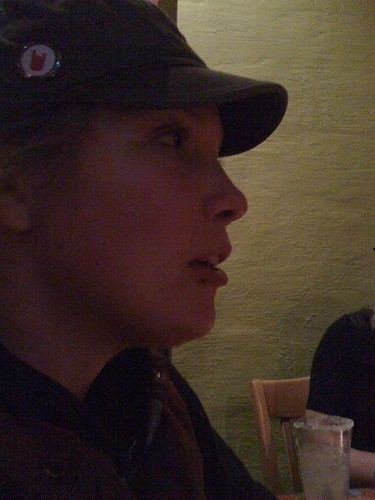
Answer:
[2,0,287,158]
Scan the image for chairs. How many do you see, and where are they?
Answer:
[252,376,310,494]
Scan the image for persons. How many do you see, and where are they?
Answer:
[305,307,375,484]
[0,0,288,499]
